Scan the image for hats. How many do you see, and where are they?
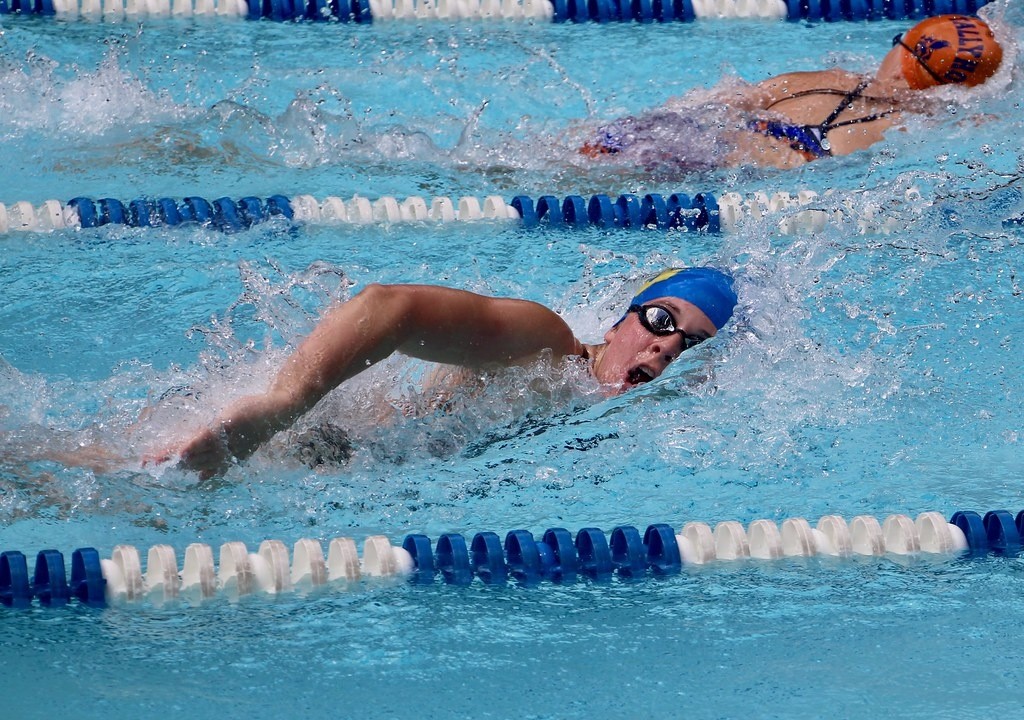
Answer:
[611,266,738,331]
[902,14,1003,90]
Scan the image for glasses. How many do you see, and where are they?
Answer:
[629,304,705,352]
[892,33,946,86]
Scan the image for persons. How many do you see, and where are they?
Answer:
[177,265,740,492]
[764,15,1004,168]
